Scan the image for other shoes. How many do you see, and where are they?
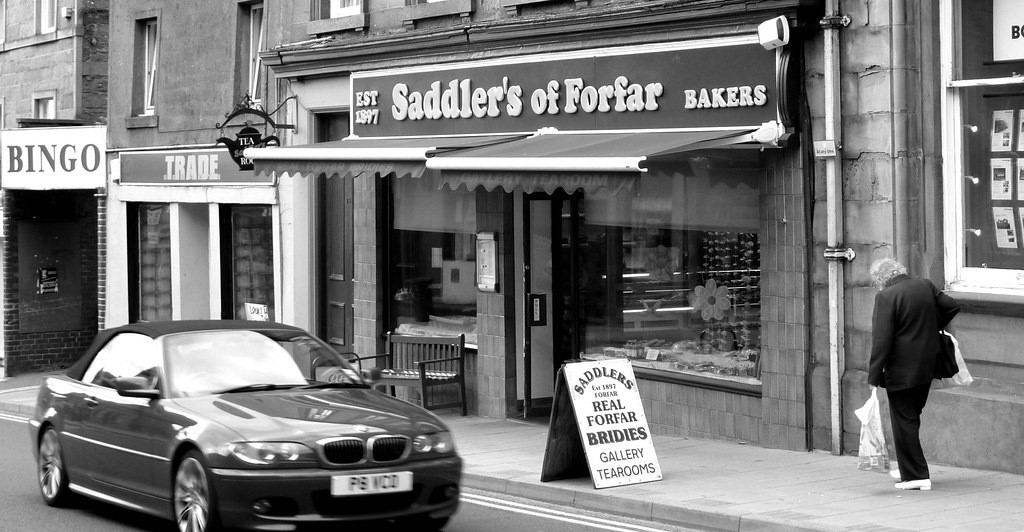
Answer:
[889,468,901,481]
[894,478,932,491]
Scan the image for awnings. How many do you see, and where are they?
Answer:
[245,126,760,199]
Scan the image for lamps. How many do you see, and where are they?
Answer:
[110,158,119,186]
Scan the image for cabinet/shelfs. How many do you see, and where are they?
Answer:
[141,223,171,321]
[234,212,273,322]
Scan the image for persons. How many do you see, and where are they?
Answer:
[867,257,961,491]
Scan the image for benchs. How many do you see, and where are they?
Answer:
[344,330,467,416]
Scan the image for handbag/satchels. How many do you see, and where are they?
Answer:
[930,329,959,380]
[853,386,893,474]
[928,329,973,392]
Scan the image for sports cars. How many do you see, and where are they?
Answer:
[30,319,464,531]
